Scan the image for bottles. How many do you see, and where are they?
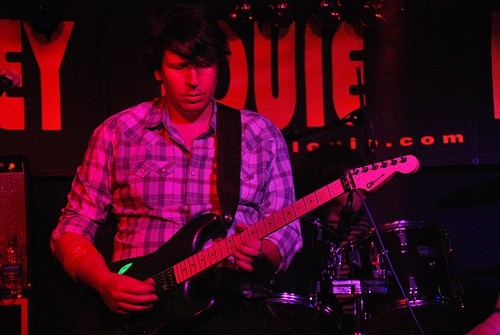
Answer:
[2,233,23,299]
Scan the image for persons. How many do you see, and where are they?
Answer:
[331,154,419,233]
[50,3,302,335]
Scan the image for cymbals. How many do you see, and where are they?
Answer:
[438,177,500,207]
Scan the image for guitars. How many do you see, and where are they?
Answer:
[72,153,420,335]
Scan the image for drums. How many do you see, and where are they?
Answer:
[343,220,465,321]
[254,215,347,335]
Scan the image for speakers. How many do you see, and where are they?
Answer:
[0,157,30,289]
